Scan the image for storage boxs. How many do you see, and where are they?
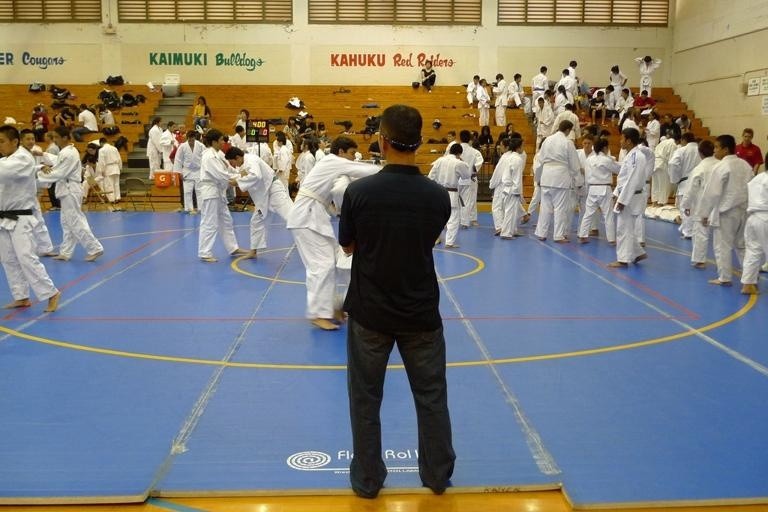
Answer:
[154,171,171,188]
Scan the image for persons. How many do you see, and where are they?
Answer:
[422,60,437,93]
[285,137,382,330]
[148,92,351,261]
[1,103,123,313]
[467,56,679,256]
[339,105,452,498]
[670,127,768,294]
[428,130,483,247]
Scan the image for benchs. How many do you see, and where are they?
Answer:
[39,188,53,211]
[0,84,164,176]
[89,185,108,203]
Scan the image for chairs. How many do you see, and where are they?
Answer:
[124,178,155,212]
[87,178,117,212]
